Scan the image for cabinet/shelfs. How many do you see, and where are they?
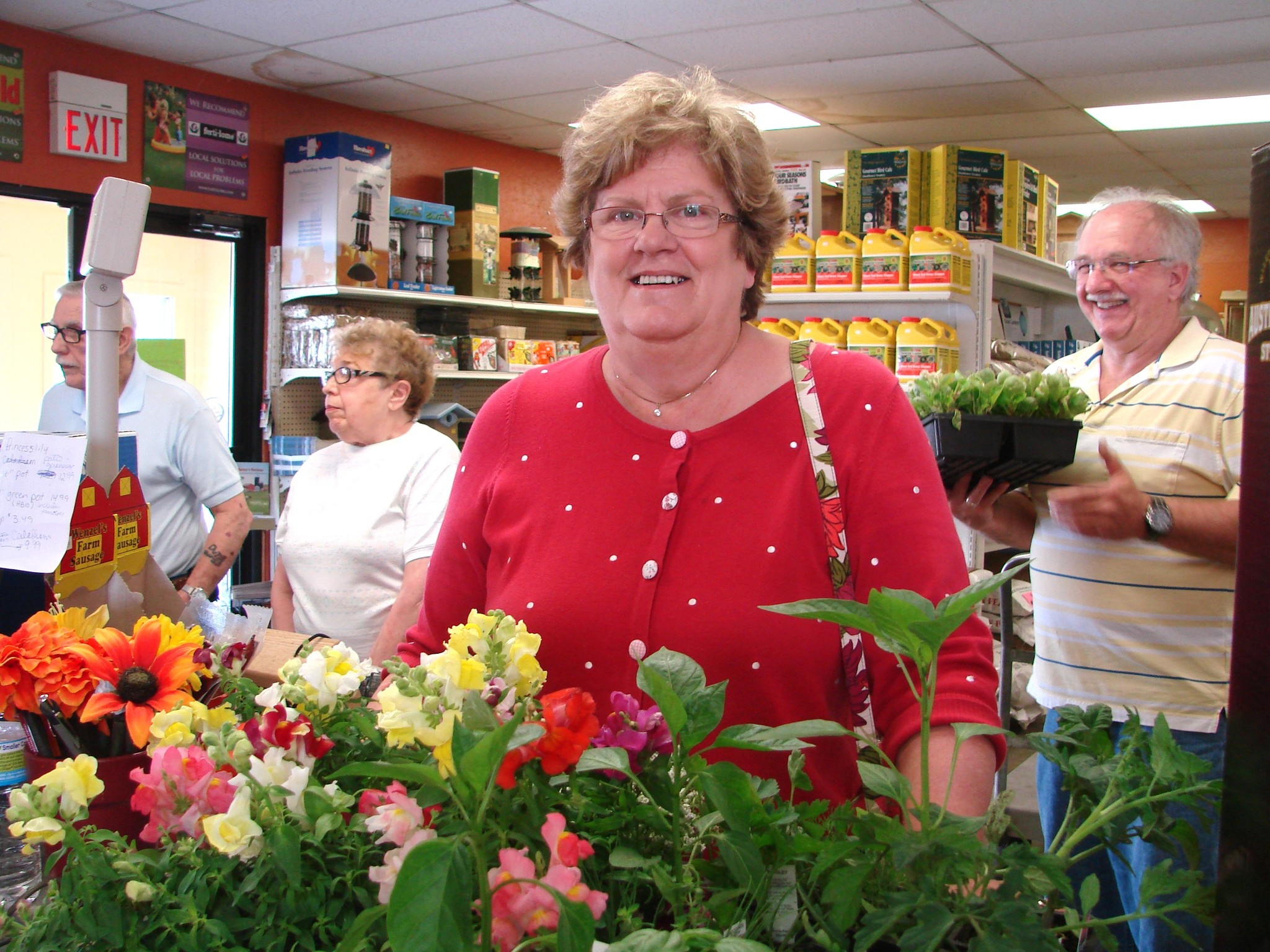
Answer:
[270,247,602,591]
[969,239,1096,571]
[759,252,985,565]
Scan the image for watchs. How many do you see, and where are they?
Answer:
[181,584,207,600]
[1144,497,1172,542]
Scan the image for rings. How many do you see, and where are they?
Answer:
[966,497,978,507]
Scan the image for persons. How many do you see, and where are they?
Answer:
[951,187,1246,952]
[38,280,253,606]
[370,73,1009,894]
[271,319,461,671]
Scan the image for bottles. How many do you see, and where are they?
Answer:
[748,226,977,384]
[0,713,41,915]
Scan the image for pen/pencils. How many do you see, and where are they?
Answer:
[49,699,135,759]
[23,710,54,758]
[39,694,97,759]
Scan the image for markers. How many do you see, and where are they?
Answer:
[18,712,39,758]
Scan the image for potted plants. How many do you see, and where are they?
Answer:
[912,368,1095,497]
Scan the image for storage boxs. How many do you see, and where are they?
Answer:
[281,130,591,374]
[991,299,1095,364]
[843,146,1062,260]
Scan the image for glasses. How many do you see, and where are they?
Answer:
[583,204,752,241]
[324,367,402,384]
[1065,256,1176,279]
[41,322,86,344]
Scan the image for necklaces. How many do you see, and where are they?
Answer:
[614,323,743,418]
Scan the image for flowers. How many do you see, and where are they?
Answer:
[0,560,1227,952]
